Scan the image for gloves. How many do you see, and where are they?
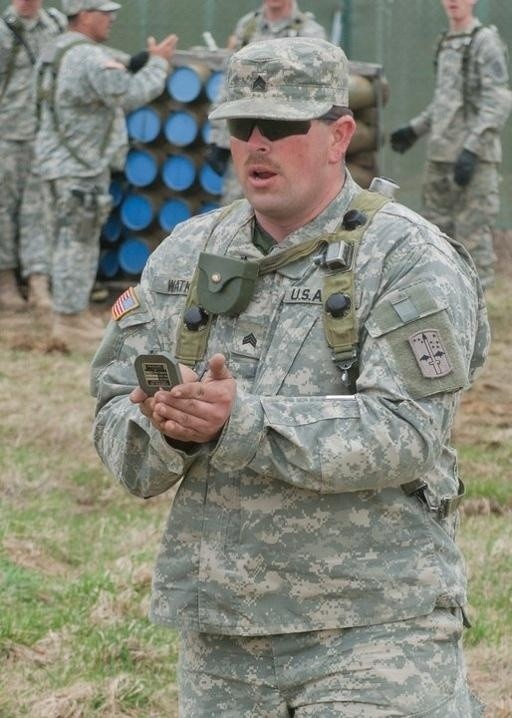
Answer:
[390,127,417,154]
[454,149,477,185]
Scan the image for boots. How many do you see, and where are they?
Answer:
[0,270,26,310]
[27,273,53,310]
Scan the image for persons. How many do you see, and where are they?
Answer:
[389,1,512,290]
[19,0,179,360]
[88,38,493,718]
[220,0,328,208]
[1,0,70,312]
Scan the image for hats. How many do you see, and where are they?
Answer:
[61,0,121,15]
[208,37,351,122]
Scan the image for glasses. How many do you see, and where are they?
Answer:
[226,118,310,143]
